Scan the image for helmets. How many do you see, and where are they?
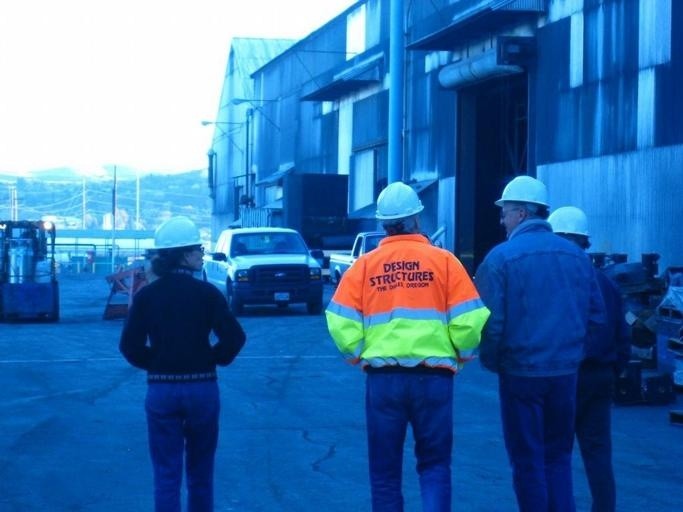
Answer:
[144,216,206,251]
[494,175,551,210]
[374,181,425,220]
[547,206,591,239]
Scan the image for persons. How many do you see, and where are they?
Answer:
[469,173,609,512]
[544,203,630,511]
[323,180,491,511]
[117,213,247,511]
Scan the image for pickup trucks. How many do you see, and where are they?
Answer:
[329,232,450,306]
[201,227,323,314]
